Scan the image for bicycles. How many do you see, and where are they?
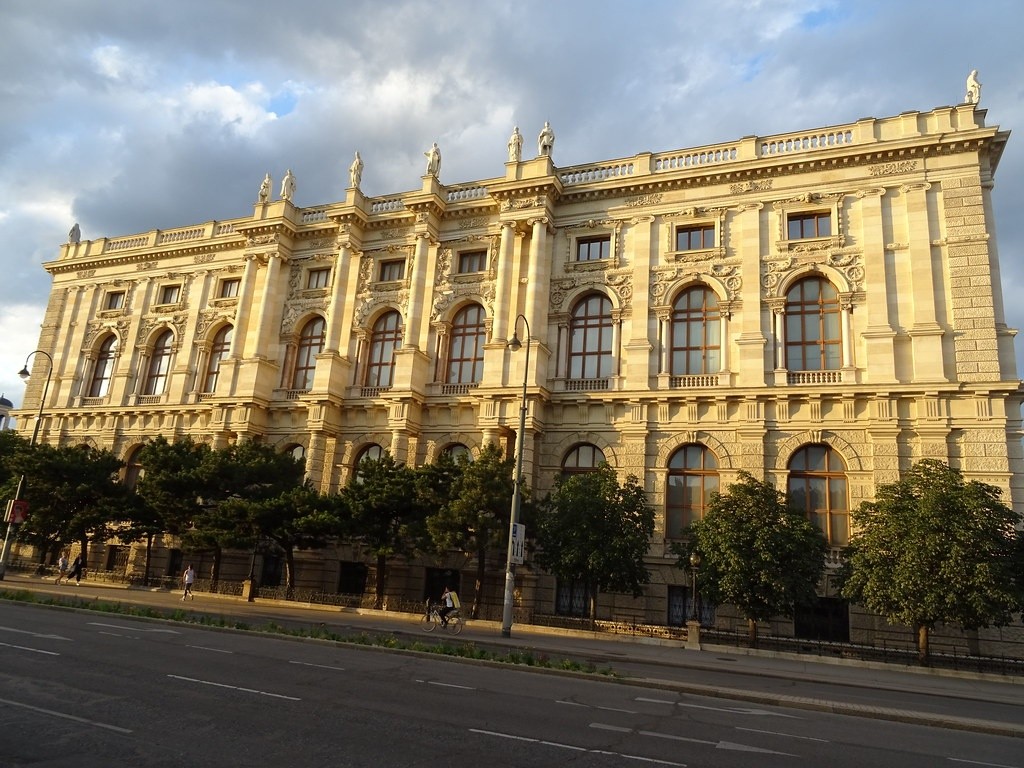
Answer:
[419,597,462,636]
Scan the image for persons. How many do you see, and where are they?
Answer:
[66,553,83,586]
[54,555,68,585]
[441,585,454,627]
[180,563,197,601]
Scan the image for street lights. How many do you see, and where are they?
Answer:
[501,315,530,637]
[682,548,702,650]
[0,350,53,582]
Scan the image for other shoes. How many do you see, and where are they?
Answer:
[441,621,448,629]
[55,580,61,586]
[66,578,69,581]
[75,581,79,586]
[180,598,185,602]
[190,595,194,601]
